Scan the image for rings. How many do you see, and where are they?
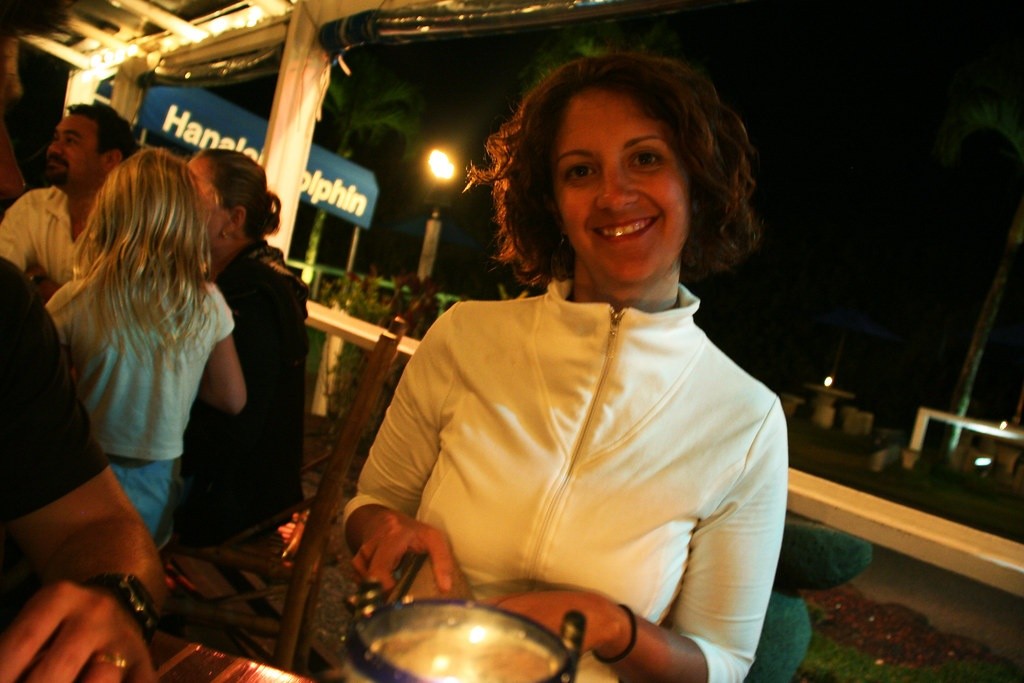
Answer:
[94,653,129,674]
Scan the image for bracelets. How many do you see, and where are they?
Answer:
[591,604,637,664]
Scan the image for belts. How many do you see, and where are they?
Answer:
[107,450,153,468]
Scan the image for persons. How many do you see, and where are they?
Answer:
[339,53,788,683]
[0,0,309,683]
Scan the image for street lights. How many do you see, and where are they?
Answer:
[416,146,456,282]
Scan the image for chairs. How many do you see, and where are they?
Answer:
[164,317,410,674]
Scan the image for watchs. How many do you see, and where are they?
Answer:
[80,572,157,646]
